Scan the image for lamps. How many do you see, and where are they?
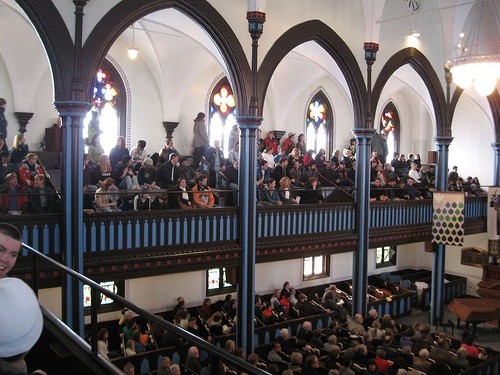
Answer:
[127,19,139,60]
[445,2,500,97]
[409,5,420,38]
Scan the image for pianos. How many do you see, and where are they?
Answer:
[448,298,500,338]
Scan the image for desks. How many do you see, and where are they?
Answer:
[448,297,500,340]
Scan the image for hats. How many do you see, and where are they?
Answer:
[0,278,44,358]
[288,132,296,137]
[144,158,153,165]
[329,285,336,290]
[4,173,17,183]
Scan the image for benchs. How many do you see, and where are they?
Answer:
[1,151,488,217]
[79,269,500,375]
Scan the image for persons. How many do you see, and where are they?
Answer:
[0,98,486,214]
[97,282,494,375]
[0,222,46,375]
